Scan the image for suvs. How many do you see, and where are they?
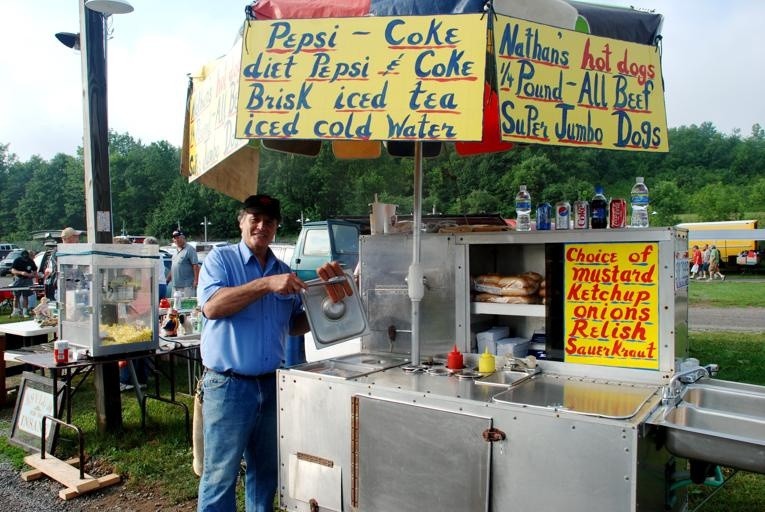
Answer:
[0,248,31,277]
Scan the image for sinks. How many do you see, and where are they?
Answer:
[678,377,765,424]
[644,401,765,473]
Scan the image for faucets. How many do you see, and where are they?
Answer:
[662,366,709,405]
[677,362,721,393]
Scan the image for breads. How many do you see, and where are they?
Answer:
[474,270,545,305]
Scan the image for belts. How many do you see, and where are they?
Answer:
[205,368,272,380]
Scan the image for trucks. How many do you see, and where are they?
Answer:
[672,219,759,272]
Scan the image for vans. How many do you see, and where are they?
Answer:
[291,221,358,290]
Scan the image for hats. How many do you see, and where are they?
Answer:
[61,227,82,239]
[171,230,184,238]
[243,194,280,218]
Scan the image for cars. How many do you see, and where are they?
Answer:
[0,243,18,249]
[190,241,227,263]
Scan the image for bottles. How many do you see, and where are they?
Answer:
[631,177,650,228]
[447,344,463,368]
[477,347,496,372]
[173,291,182,310]
[515,184,531,231]
[54,339,69,364]
[591,186,607,228]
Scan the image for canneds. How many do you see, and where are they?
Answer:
[536,202,552,230]
[573,201,589,229]
[609,198,626,229]
[554,201,571,229]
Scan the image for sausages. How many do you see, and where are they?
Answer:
[317,267,339,303]
[330,261,353,296]
[322,262,345,299]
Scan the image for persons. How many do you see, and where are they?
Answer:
[689,243,728,283]
[7,228,200,318]
[197,192,312,511]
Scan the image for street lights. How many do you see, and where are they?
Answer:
[200,217,213,242]
[296,211,311,227]
[54,0,135,432]
[647,208,658,220]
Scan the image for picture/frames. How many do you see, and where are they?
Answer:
[7,371,67,456]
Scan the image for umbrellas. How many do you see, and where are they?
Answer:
[185,0,670,375]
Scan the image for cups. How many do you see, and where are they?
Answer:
[368,203,399,235]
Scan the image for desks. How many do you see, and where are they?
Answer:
[0,286,200,446]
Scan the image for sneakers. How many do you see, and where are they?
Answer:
[9,311,31,318]
[119,382,148,393]
